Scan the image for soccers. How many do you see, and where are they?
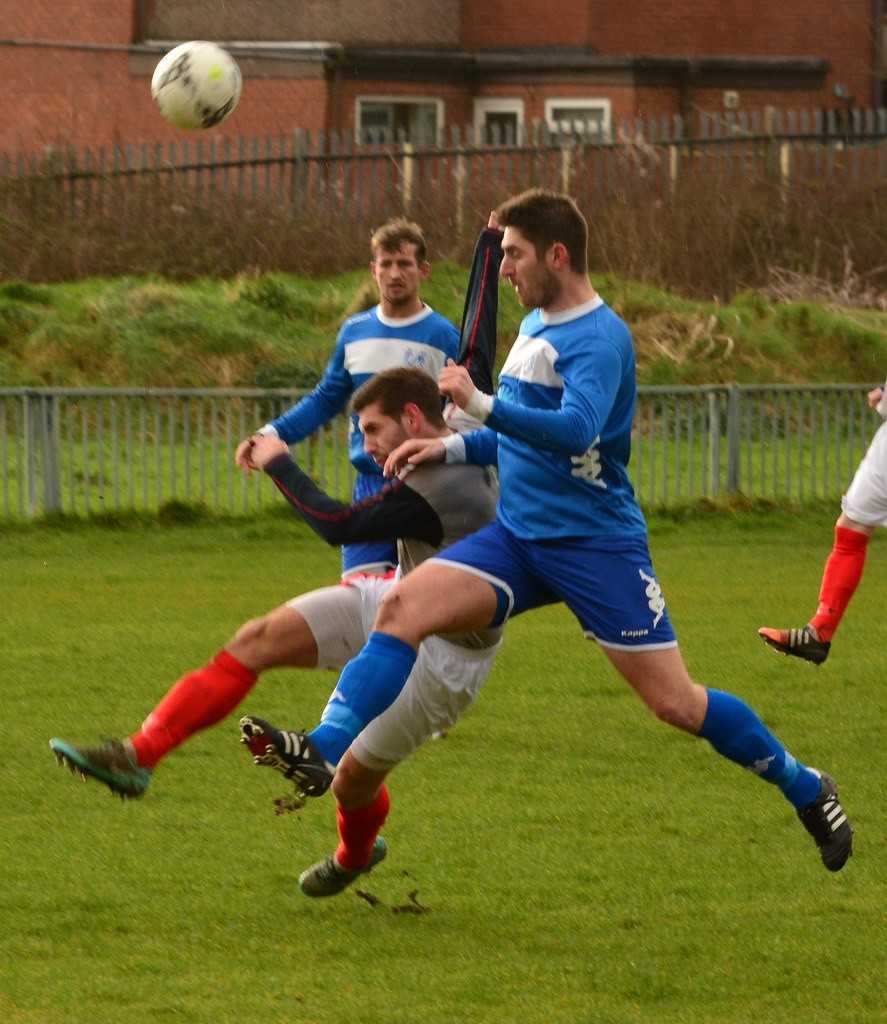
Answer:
[148,38,242,133]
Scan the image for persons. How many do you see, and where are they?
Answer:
[758,381,887,664]
[233,217,462,577]
[49,366,504,898]
[239,190,854,873]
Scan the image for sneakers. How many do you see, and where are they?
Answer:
[238,715,335,796]
[796,766,853,872]
[299,834,386,896]
[47,737,152,797]
[758,626,831,667]
